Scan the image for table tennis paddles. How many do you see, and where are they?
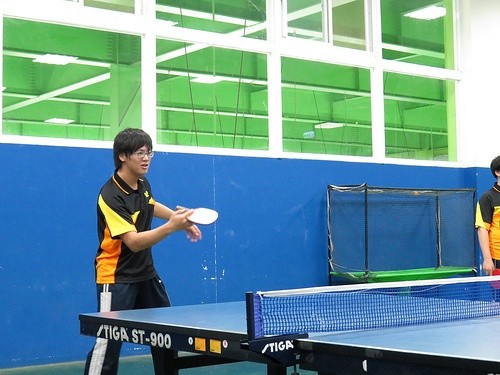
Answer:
[489,268,500,288]
[176,204,219,226]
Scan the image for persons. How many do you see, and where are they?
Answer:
[474,156,500,302]
[82,128,202,375]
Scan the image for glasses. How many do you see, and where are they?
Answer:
[133,151,154,159]
